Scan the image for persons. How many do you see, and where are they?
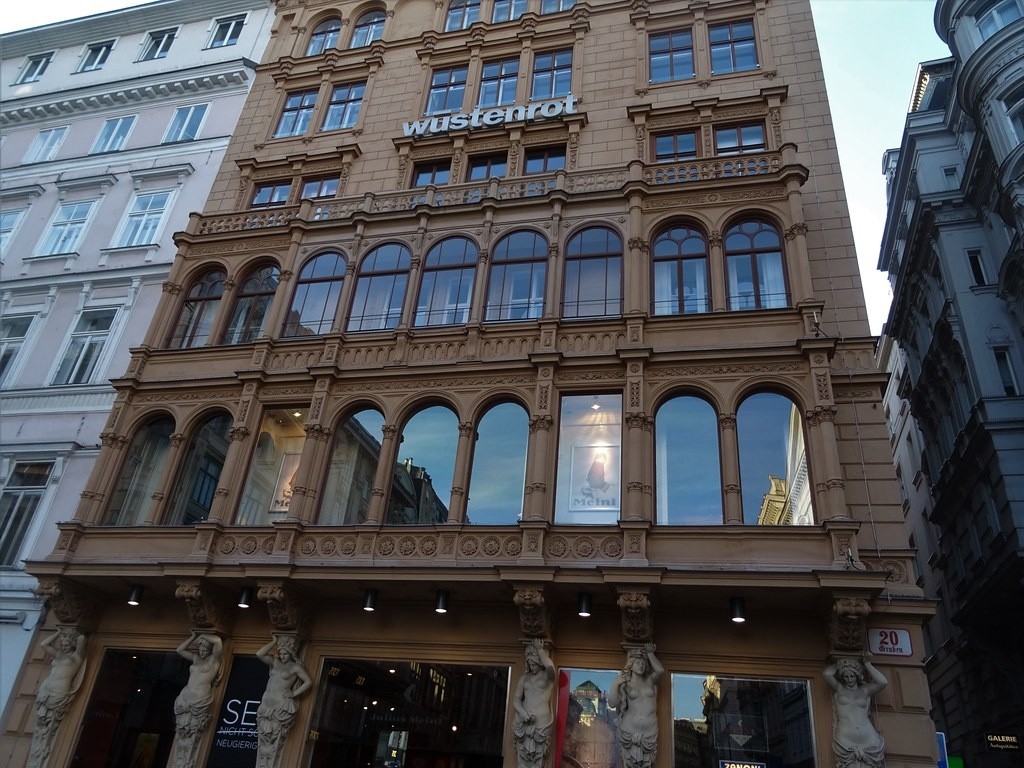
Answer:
[173,631,225,738]
[35,627,88,726]
[609,644,665,768]
[511,636,560,768]
[822,651,888,768]
[255,635,313,738]
